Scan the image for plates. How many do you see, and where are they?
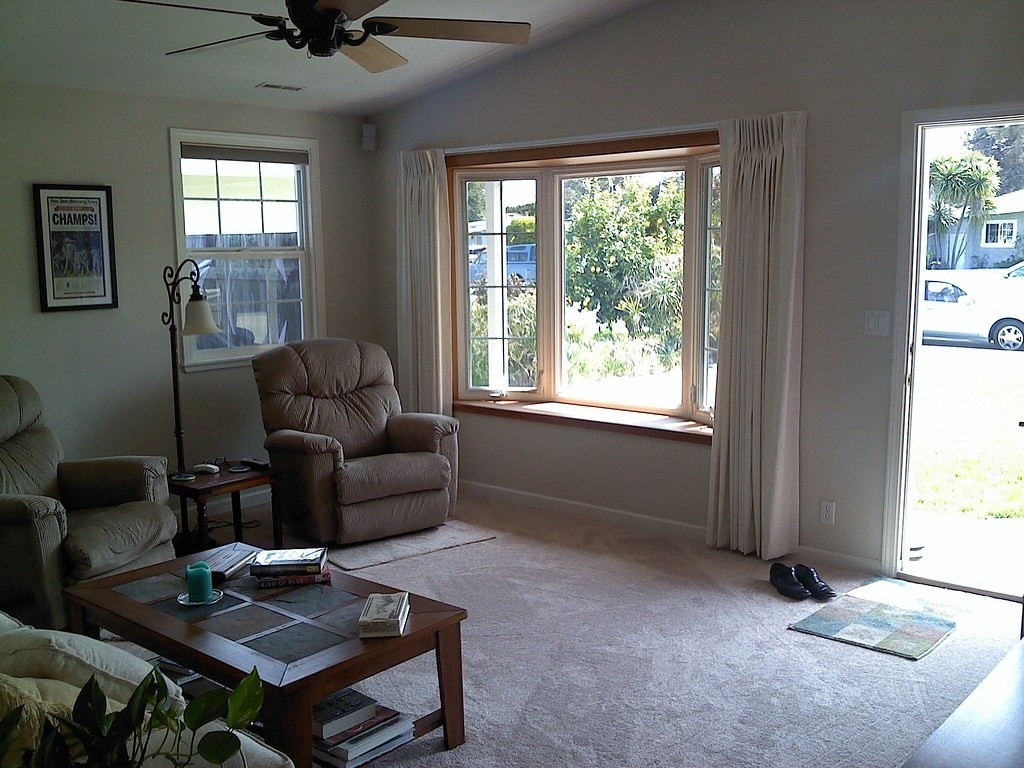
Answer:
[176,588,223,606]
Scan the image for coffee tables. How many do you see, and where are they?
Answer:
[67,541,470,767]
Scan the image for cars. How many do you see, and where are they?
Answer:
[923,270,1024,351]
[1001,261,1024,283]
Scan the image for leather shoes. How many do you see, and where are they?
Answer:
[769,561,812,600]
[793,563,839,600]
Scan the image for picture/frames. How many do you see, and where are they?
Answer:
[32,183,120,313]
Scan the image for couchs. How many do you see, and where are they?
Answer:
[0,374,178,635]
[0,609,296,767]
[249,338,460,543]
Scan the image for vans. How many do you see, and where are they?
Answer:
[468,243,536,285]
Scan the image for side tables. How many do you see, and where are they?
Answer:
[167,461,285,549]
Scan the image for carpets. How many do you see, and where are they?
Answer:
[326,519,498,571]
[787,573,959,660]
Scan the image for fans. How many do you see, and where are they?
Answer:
[120,0,532,74]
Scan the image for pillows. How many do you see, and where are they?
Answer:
[0,672,151,754]
[1,625,187,720]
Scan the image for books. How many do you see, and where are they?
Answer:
[310,688,416,768]
[186,549,255,580]
[156,657,226,703]
[250,547,331,589]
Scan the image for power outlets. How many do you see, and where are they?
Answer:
[818,500,835,526]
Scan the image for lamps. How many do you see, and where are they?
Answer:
[161,259,223,558]
